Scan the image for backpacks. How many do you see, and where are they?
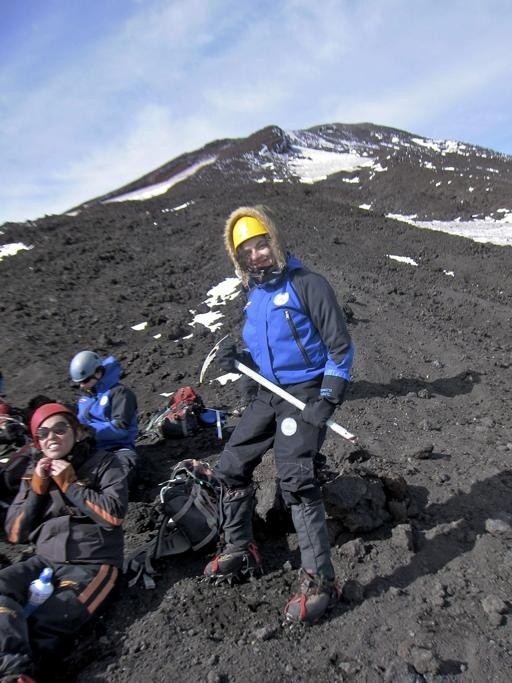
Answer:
[149,457,224,554]
[145,386,205,445]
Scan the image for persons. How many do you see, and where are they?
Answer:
[202,201,356,625]
[68,348,140,485]
[0,398,127,681]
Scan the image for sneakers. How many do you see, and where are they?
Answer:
[282,578,340,624]
[202,545,266,587]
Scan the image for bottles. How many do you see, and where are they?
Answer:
[22,567,56,618]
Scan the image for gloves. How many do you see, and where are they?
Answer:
[299,396,336,430]
[215,343,238,372]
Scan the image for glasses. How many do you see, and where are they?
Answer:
[34,422,75,439]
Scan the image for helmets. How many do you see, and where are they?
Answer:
[232,215,271,250]
[68,350,105,383]
[30,402,80,454]
